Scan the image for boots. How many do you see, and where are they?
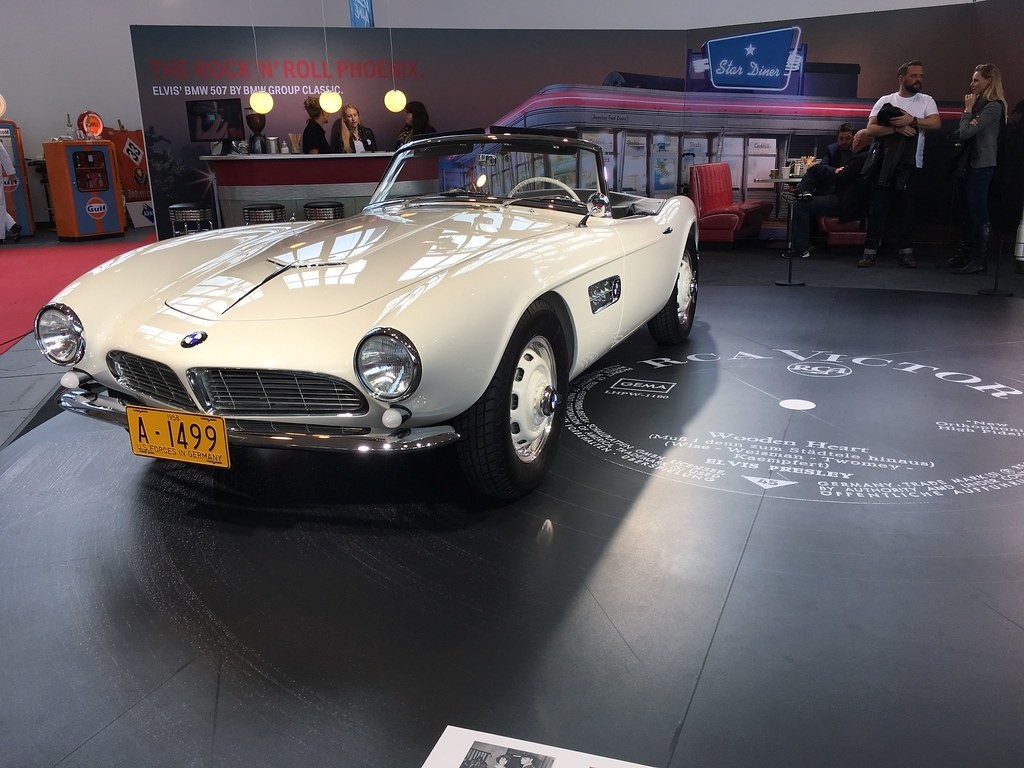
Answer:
[952,221,992,274]
[944,225,971,271]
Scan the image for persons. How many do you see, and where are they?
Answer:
[303,95,437,154]
[781,59,1024,274]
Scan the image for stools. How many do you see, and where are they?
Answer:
[168,203,213,238]
[242,204,286,226]
[304,201,344,221]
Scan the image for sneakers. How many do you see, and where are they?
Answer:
[781,247,810,258]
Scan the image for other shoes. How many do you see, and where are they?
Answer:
[858,253,877,266]
[899,252,916,268]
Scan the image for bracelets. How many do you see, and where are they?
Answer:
[964,110,971,113]
[910,117,917,127]
[893,128,896,134]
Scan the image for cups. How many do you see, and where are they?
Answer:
[782,167,791,179]
[792,164,801,175]
[210,141,223,155]
[771,169,779,179]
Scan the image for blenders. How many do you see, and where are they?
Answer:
[244,108,266,154]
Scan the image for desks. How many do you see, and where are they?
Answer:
[754,177,803,259]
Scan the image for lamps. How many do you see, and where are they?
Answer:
[318,27,343,113]
[249,26,274,114]
[384,28,407,113]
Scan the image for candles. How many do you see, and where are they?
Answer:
[67,113,70,123]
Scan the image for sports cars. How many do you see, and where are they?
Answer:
[33,127,701,503]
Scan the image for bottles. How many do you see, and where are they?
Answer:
[65,113,73,136]
[76,154,103,191]
[281,141,289,154]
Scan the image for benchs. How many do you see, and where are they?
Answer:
[690,162,775,246]
[818,216,870,245]
[499,189,649,211]
